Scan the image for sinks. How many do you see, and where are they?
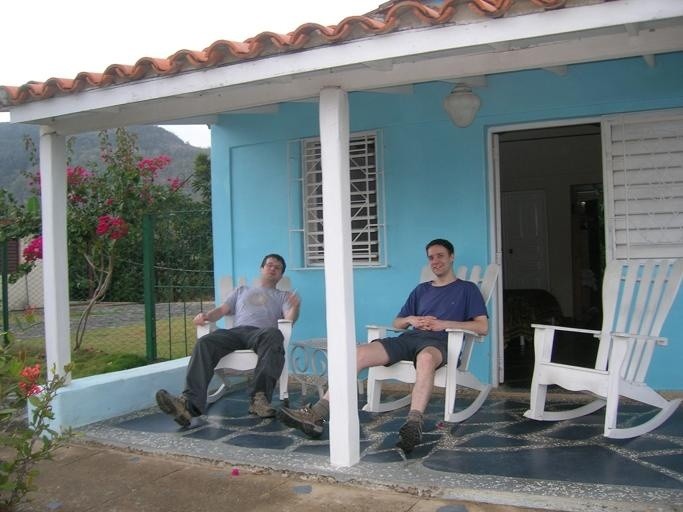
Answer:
[503,287,571,349]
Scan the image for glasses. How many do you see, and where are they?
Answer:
[443,80,480,130]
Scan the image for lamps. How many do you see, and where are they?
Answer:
[522,258,683,446]
[193,273,297,412]
[360,262,501,424]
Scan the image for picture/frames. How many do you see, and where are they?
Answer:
[291,338,329,405]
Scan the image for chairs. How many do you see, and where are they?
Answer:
[248,393,276,418]
[396,410,424,454]
[157,389,193,427]
[279,402,325,440]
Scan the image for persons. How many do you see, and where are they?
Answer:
[275,238,490,455]
[156,254,302,428]
